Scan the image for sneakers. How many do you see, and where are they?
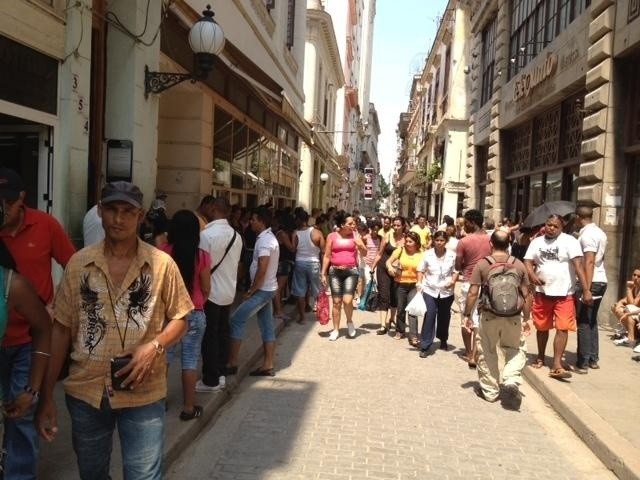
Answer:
[328,329,340,341]
[477,387,498,402]
[441,339,448,349]
[275,302,316,325]
[572,326,640,374]
[463,349,479,369]
[194,379,221,393]
[420,344,436,359]
[218,375,226,389]
[248,368,275,377]
[393,332,405,340]
[499,382,522,410]
[409,336,422,347]
[346,320,357,337]
[179,405,204,420]
[222,365,238,375]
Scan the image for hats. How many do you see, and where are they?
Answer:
[100,180,144,209]
[151,198,167,211]
[0,166,26,200]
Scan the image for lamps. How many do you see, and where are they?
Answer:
[497,40,552,76]
[320,172,329,187]
[144,5,226,102]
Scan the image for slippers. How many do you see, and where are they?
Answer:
[547,367,573,380]
[531,356,545,369]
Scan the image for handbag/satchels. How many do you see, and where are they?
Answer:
[392,266,402,278]
[357,280,373,312]
[365,291,378,313]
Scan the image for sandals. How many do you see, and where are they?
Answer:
[389,319,396,331]
[376,326,387,335]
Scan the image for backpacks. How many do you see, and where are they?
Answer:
[482,255,526,318]
[316,288,330,325]
